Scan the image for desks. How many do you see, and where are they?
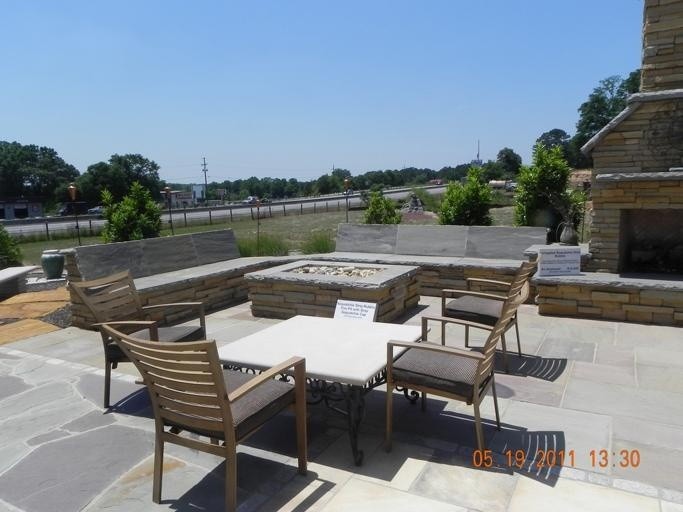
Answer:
[217,315,431,466]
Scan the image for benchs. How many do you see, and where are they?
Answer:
[72,223,549,331]
[0,265,43,292]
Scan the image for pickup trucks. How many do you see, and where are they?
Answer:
[242,196,258,204]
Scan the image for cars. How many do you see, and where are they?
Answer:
[87,206,110,215]
[260,198,271,203]
[343,188,353,195]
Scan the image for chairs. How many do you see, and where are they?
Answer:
[385,280,531,454]
[102,325,307,512]
[68,269,206,407]
[442,260,538,372]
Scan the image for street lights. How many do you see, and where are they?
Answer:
[342,177,349,223]
[163,187,174,236]
[255,199,260,256]
[66,185,80,246]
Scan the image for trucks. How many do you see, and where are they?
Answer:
[488,180,517,192]
[59,202,87,215]
